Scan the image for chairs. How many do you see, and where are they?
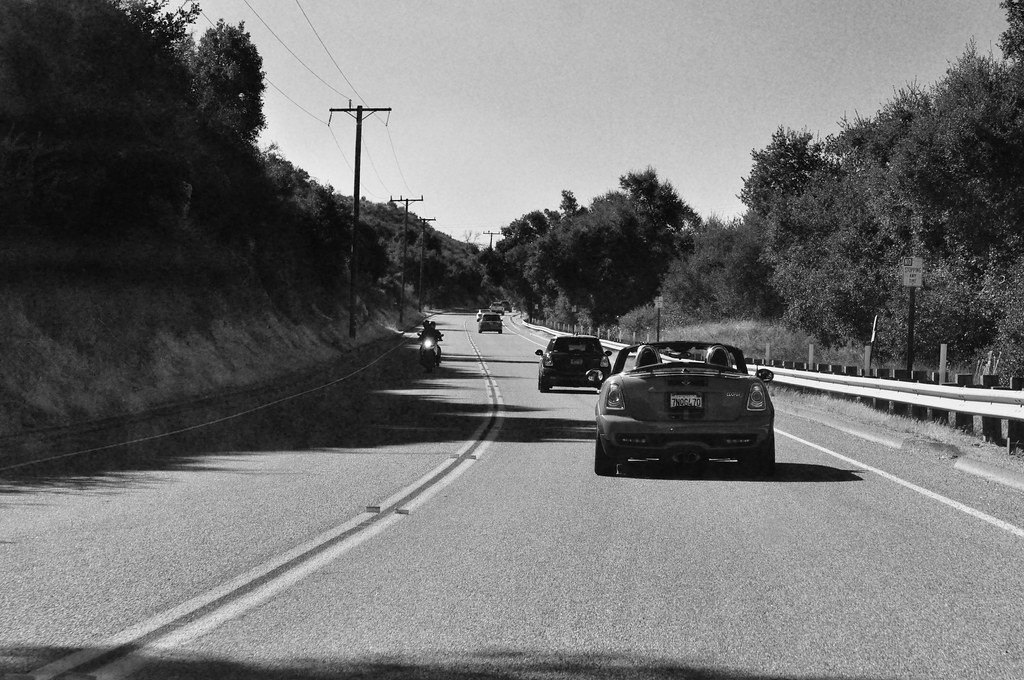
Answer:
[635,347,662,368]
[706,345,732,367]
[584,345,595,352]
[556,342,569,352]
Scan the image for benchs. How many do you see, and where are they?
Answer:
[624,361,744,374]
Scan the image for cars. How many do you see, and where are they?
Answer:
[535,336,612,391]
[477,312,503,334]
[476,300,513,322]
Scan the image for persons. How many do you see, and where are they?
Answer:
[417,320,442,363]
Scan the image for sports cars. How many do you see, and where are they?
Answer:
[586,340,777,478]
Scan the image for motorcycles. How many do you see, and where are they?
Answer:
[416,332,445,372]
